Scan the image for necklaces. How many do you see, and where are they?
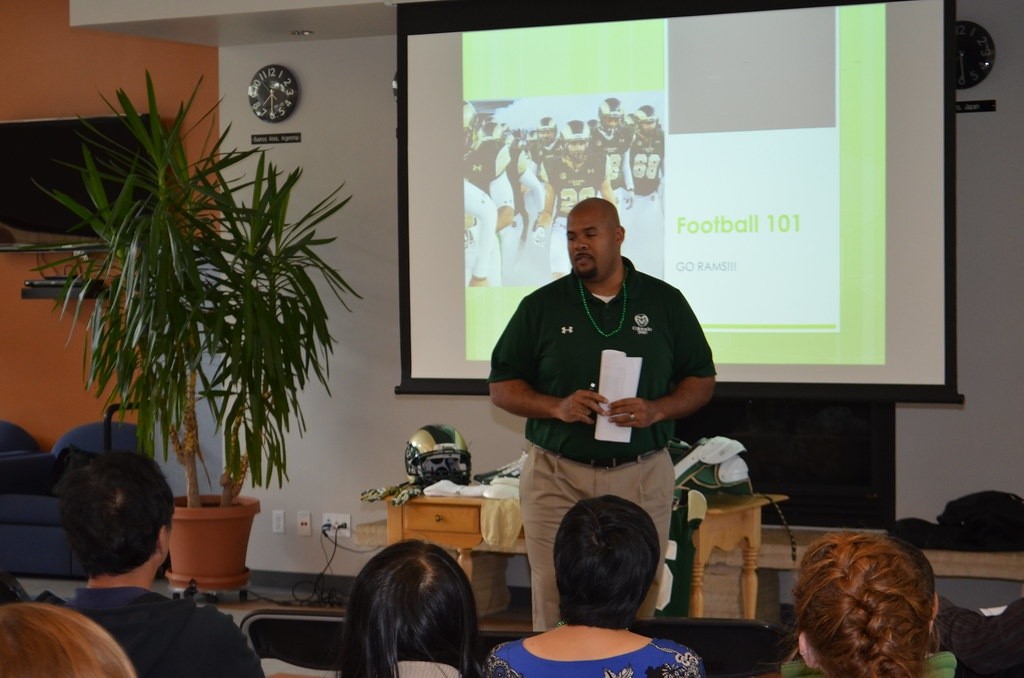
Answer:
[578,280,627,338]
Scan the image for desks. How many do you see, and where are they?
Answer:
[383,494,787,618]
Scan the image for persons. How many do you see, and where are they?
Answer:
[483,494,706,678]
[488,198,717,633]
[461,97,664,287]
[780,531,956,678]
[341,539,484,678]
[32,452,266,678]
[935,588,1024,678]
[0,568,135,678]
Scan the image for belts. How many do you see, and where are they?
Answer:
[531,441,666,468]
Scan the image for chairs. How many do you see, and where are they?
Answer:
[0,417,780,678]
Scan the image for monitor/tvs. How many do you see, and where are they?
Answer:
[0,113,162,253]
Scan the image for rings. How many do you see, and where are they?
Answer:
[630,413,635,421]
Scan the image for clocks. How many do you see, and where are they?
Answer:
[955,20,996,88]
[248,64,298,122]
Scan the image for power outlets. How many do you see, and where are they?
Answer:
[272,510,284,534]
[322,514,350,539]
[298,511,312,536]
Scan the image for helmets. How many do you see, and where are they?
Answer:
[537,116,558,147]
[625,113,641,128]
[635,105,658,134]
[462,95,536,157]
[562,121,593,159]
[405,424,472,485]
[599,99,625,134]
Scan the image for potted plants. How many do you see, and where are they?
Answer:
[16,66,364,603]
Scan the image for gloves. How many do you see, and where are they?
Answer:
[392,484,421,506]
[361,481,409,503]
